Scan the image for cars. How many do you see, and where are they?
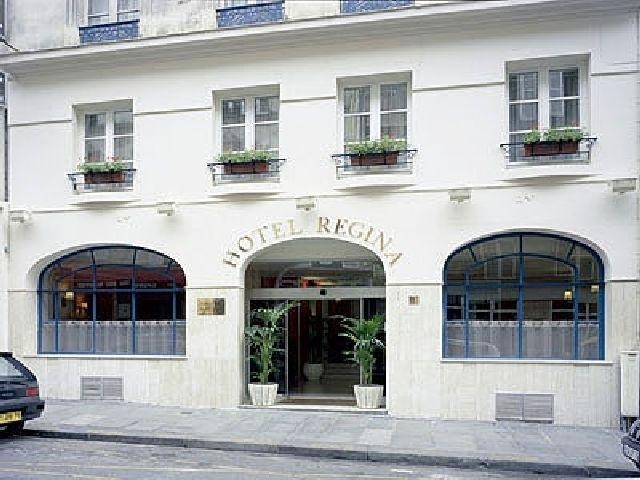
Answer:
[0,350,47,439]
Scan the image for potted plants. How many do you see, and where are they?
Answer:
[522,127,584,155]
[347,136,404,165]
[75,158,125,183]
[215,151,271,173]
[339,313,385,409]
[244,300,302,403]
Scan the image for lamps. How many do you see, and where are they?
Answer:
[563,287,572,301]
[61,292,73,309]
